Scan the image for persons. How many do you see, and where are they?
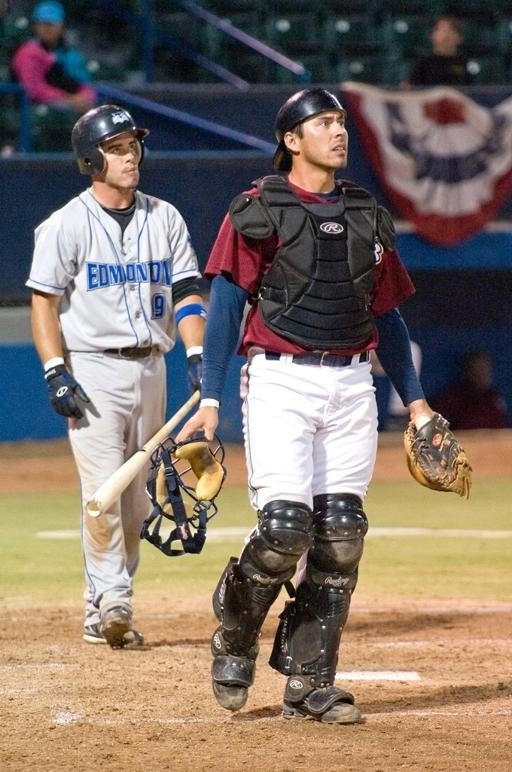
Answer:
[175,88,469,724]
[24,105,208,650]
[9,0,97,111]
[400,16,479,90]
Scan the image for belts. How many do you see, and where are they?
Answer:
[104,346,152,359]
[263,348,371,369]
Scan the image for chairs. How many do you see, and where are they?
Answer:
[0,1,512,150]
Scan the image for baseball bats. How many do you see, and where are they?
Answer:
[86,390,202,519]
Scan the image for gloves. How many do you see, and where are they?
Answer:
[186,346,204,392]
[44,364,91,419]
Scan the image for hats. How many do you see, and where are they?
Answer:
[31,1,65,26]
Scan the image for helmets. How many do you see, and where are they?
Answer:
[71,105,150,177]
[273,87,347,171]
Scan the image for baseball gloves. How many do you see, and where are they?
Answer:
[404,413,472,499]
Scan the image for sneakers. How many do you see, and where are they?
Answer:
[283,689,361,725]
[82,605,145,650]
[213,683,249,710]
